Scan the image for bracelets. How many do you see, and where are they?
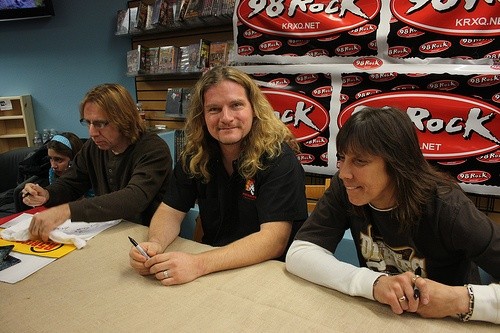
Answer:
[457,284,474,323]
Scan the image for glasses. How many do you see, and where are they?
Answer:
[80,117,110,129]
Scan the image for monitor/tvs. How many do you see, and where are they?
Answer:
[0,0,54,21]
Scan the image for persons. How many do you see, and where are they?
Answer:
[47,132,95,200]
[21,83,172,244]
[286,108,500,325]
[129,64,308,286]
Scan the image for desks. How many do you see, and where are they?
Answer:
[0,202,500,333]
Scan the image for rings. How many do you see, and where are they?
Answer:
[412,275,420,283]
[164,270,169,279]
[398,296,406,302]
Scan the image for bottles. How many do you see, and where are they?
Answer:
[33,130,42,147]
[49,129,57,140]
[42,128,49,144]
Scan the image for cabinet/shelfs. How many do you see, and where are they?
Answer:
[121,13,233,79]
[0,95,36,154]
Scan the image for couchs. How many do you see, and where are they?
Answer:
[0,147,49,218]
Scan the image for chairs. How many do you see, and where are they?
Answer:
[194,179,330,244]
[159,130,199,241]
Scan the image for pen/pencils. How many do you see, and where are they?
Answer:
[23,184,39,198]
[414,266,421,299]
[128,236,150,260]
